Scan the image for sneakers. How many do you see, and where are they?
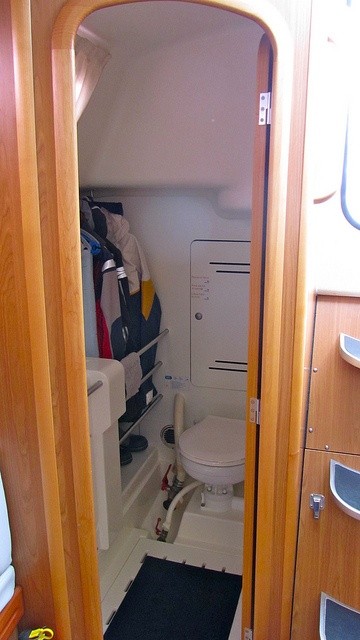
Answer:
[118,428,147,452]
[119,447,133,467]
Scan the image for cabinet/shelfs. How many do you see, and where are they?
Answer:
[287,296,359,639]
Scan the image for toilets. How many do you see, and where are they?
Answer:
[179,416,246,512]
